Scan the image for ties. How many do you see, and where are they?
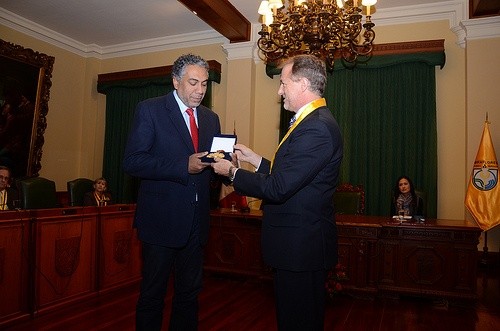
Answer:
[186,108,199,154]
[0,192,6,211]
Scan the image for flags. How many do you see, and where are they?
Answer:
[464,122,500,231]
[216,160,248,214]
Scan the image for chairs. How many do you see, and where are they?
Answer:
[334,182,364,213]
[21,176,56,208]
[66,178,93,206]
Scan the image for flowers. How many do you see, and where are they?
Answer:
[325,264,346,297]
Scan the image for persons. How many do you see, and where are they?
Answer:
[125,54,232,331]
[210,54,343,331]
[390,175,423,217]
[82,176,114,206]
[0,166,14,211]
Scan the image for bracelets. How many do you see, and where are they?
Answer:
[229,165,235,180]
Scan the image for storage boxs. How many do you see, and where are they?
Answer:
[201,133,238,163]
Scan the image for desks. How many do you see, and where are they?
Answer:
[0,202,482,331]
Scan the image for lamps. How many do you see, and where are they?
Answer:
[255,0,377,70]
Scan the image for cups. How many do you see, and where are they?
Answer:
[231,201,239,212]
[398,210,404,221]
[99,200,107,206]
